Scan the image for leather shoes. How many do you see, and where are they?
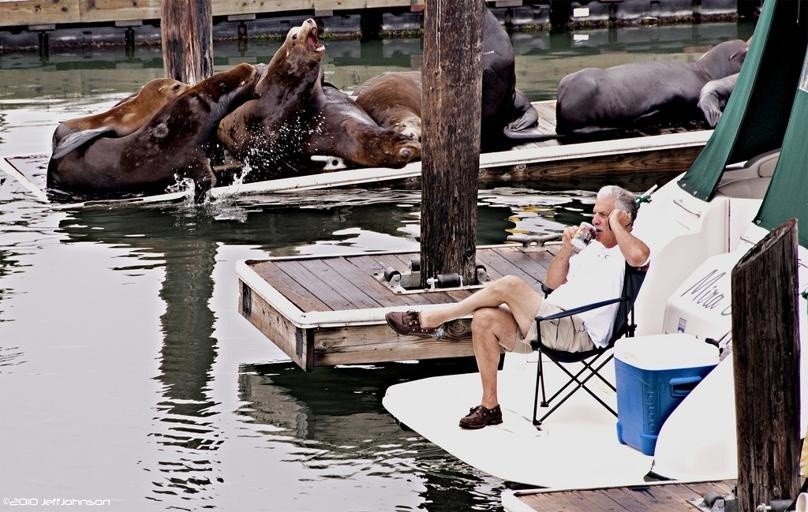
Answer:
[459,404,503,431]
[384,310,443,339]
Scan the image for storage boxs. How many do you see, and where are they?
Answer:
[612,333,720,456]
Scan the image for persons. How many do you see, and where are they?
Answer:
[386,184,652,429]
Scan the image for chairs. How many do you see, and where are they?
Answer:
[528,259,644,425]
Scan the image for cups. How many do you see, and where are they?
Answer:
[795,491,808,512]
[571,222,597,250]
